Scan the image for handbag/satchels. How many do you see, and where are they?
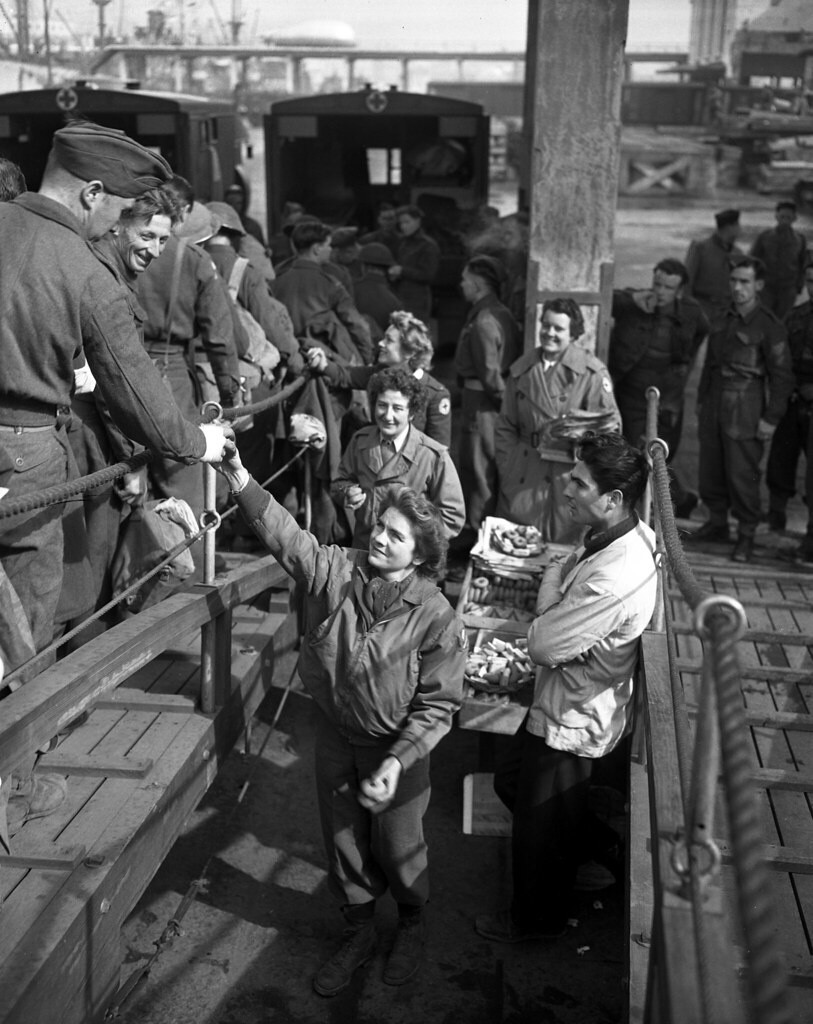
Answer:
[114,497,204,618]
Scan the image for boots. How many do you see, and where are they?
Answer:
[313,899,377,995]
[384,899,427,985]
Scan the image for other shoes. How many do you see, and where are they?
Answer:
[777,546,813,567]
[476,908,566,942]
[761,517,784,530]
[732,533,754,563]
[681,521,730,542]
[5,774,67,835]
[675,493,700,518]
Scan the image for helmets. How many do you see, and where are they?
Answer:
[180,201,221,246]
[206,201,247,239]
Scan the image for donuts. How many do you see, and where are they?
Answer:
[505,524,540,548]
[463,568,543,610]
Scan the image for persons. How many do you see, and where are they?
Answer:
[474,431,659,944]
[0,117,813,837]
[210,417,469,995]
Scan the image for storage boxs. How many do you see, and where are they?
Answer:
[454,556,534,638]
[457,629,533,740]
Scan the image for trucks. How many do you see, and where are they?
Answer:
[261,85,494,246]
[0,80,248,225]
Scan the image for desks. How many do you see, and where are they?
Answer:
[619,132,713,195]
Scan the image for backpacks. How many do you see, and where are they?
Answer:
[226,256,280,391]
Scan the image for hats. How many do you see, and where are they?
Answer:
[333,225,359,248]
[716,210,739,229]
[355,244,396,267]
[467,256,510,286]
[55,128,173,198]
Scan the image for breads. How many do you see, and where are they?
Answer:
[463,603,538,623]
[464,637,533,688]
